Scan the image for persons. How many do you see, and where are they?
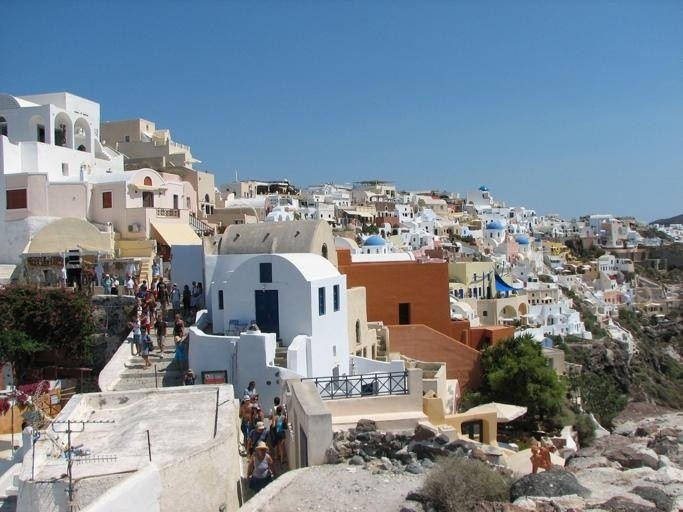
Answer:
[247,319,259,330]
[57,258,204,386]
[238,381,288,493]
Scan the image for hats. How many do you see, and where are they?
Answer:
[187,369,192,374]
[175,336,181,343]
[243,395,267,449]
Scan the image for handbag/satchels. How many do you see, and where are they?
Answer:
[148,340,153,350]
[266,468,273,482]
